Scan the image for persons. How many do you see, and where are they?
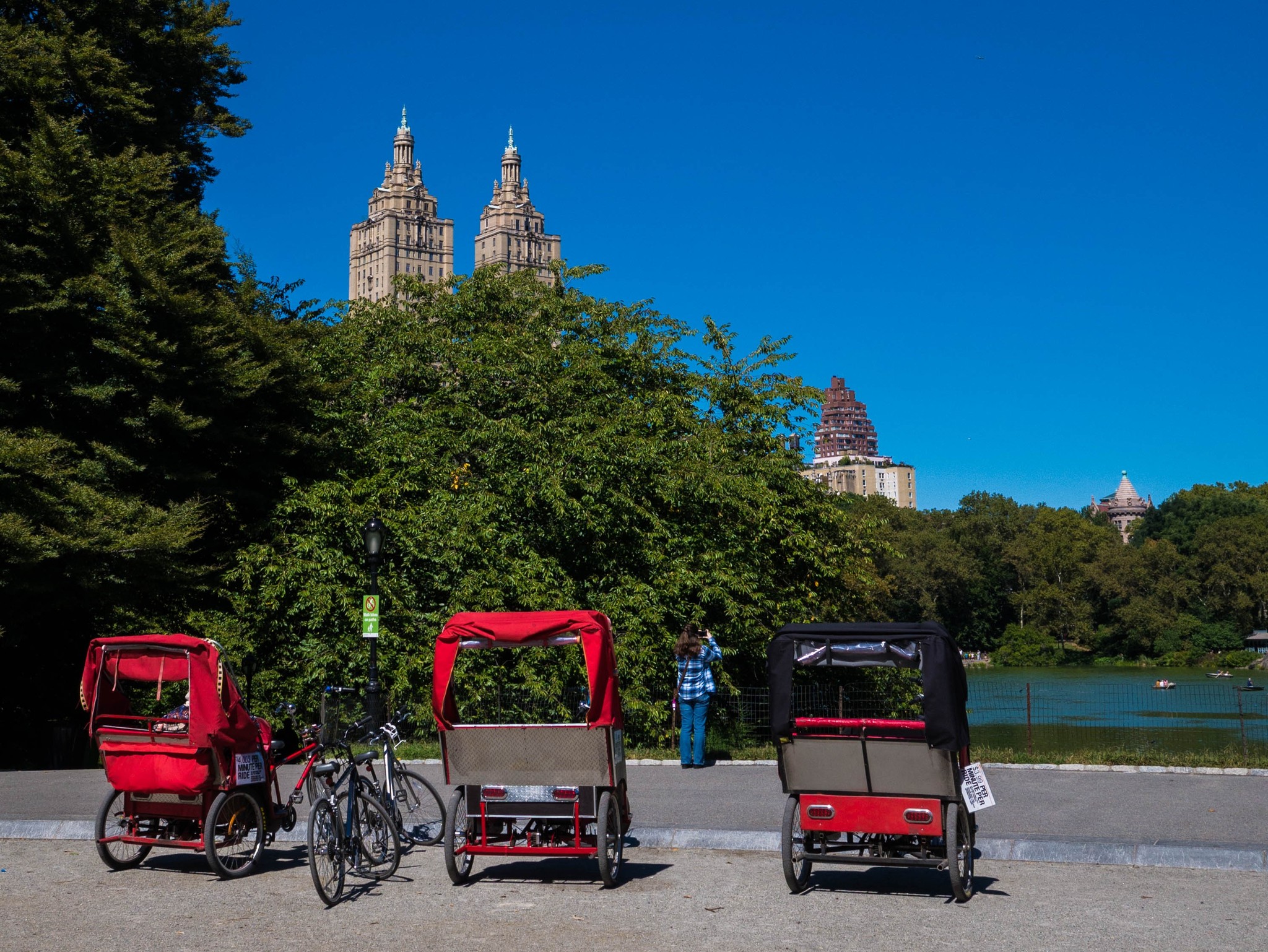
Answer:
[984,651,988,661]
[673,623,722,768]
[1247,678,1253,687]
[1156,679,1160,687]
[1160,680,1164,687]
[1163,678,1168,686]
[977,650,981,660]
[1217,668,1221,674]
[971,652,974,661]
[959,649,962,655]
[964,652,968,663]
[968,652,971,662]
[1226,669,1229,675]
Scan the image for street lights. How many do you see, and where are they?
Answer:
[360,511,389,737]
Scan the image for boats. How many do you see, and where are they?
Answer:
[1151,682,1177,689]
[1239,685,1265,691]
[1205,673,1234,679]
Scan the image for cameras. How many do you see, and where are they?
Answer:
[699,631,707,637]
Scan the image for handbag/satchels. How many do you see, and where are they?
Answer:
[673,688,678,702]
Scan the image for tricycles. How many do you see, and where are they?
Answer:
[769,621,978,903]
[79,632,358,881]
[433,611,634,889]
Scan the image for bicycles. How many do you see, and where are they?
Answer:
[304,704,448,907]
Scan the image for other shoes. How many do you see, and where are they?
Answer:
[693,760,712,769]
[682,760,693,769]
